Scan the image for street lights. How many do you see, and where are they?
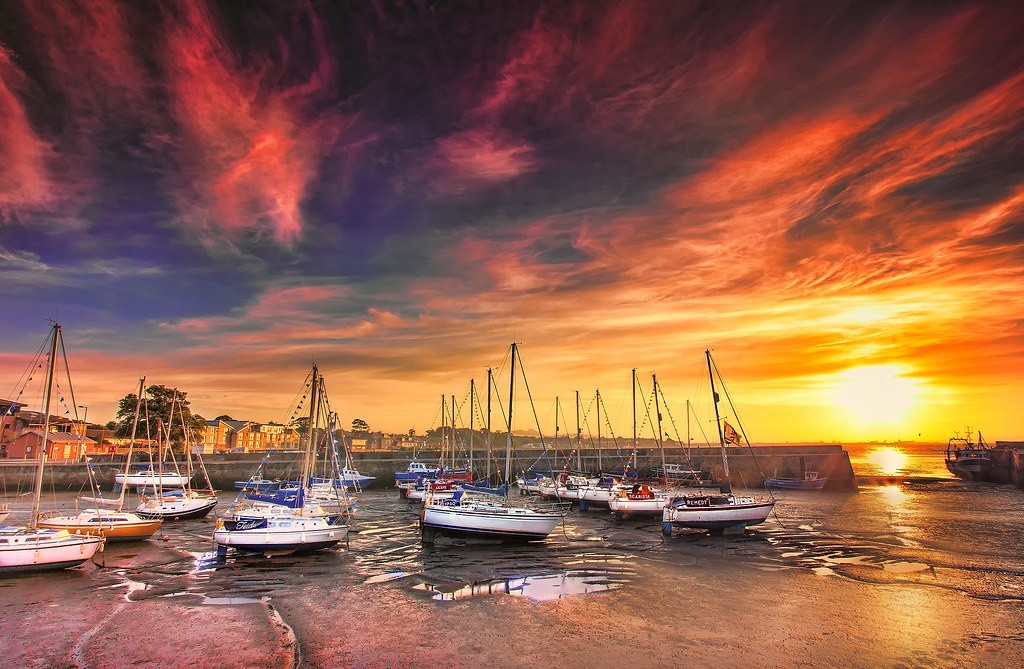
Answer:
[78,406,87,458]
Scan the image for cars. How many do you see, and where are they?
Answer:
[121,451,157,462]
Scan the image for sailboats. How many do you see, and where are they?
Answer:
[662,348,776,530]
[114,387,197,490]
[26,376,163,542]
[608,374,688,514]
[516,391,599,498]
[0,323,106,574]
[555,389,625,500]
[135,427,218,522]
[577,368,661,508]
[214,364,377,552]
[395,343,573,541]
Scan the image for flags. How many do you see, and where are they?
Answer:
[724,420,742,446]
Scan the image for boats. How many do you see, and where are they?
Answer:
[650,463,702,479]
[764,470,826,489]
[944,426,993,480]
